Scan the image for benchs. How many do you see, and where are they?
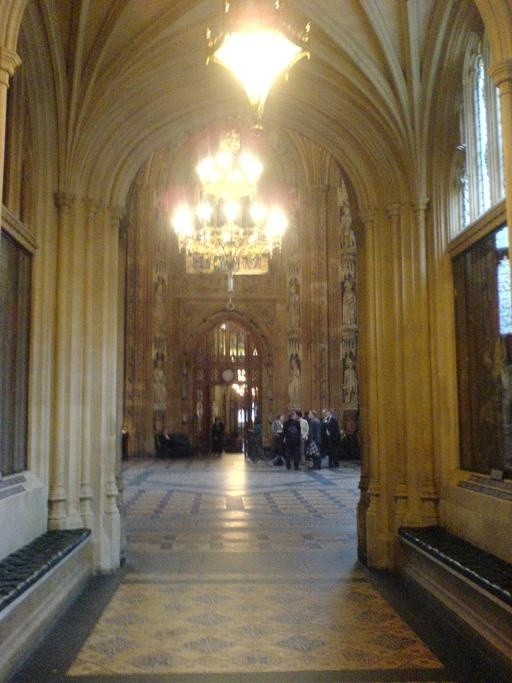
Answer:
[394,522,511,672]
[0,527,93,681]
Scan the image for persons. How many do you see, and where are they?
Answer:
[212,417,225,456]
[248,416,266,463]
[272,409,342,471]
[159,428,176,449]
